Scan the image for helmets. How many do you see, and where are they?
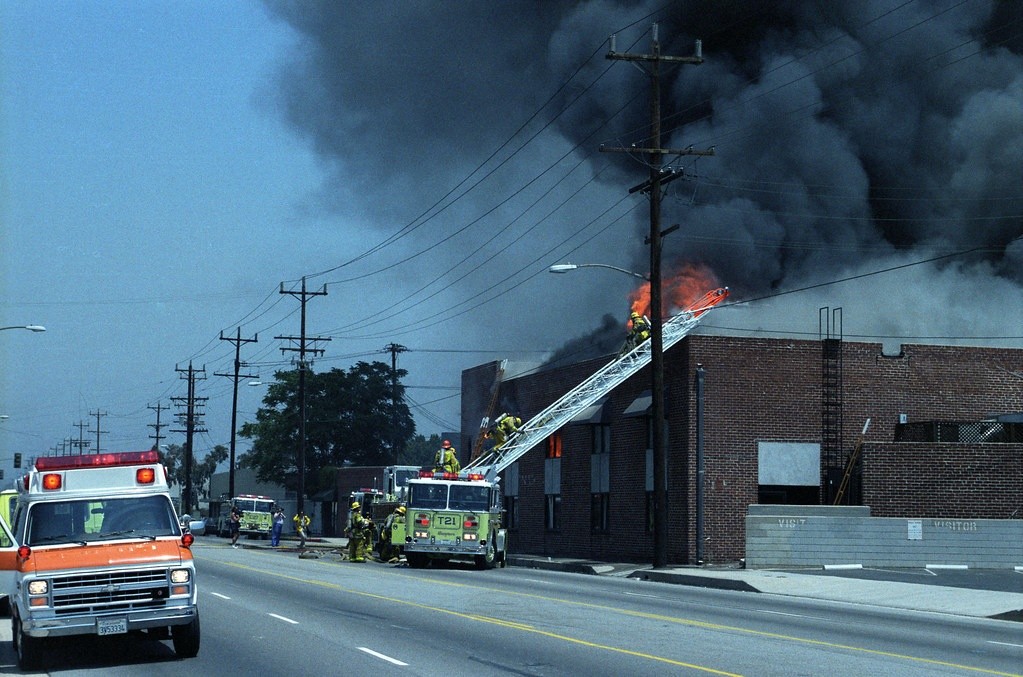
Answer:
[352,501,359,509]
[441,440,451,449]
[515,417,522,426]
[631,312,639,318]
[395,506,407,514]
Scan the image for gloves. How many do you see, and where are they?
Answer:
[517,430,524,434]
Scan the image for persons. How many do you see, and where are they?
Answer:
[293,510,310,549]
[231,507,241,548]
[349,502,373,562]
[381,506,406,561]
[490,417,524,454]
[432,441,460,473]
[119,499,156,531]
[272,507,286,547]
[184,513,191,529]
[626,312,649,358]
[465,487,486,501]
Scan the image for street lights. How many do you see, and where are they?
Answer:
[160,444,194,516]
[550,263,673,571]
[247,380,305,517]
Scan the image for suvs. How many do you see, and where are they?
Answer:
[0,452,203,672]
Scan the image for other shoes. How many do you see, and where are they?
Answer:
[231,545,238,549]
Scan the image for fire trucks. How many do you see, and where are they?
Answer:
[389,284,730,572]
[219,492,278,540]
[0,488,105,549]
[343,359,508,558]
